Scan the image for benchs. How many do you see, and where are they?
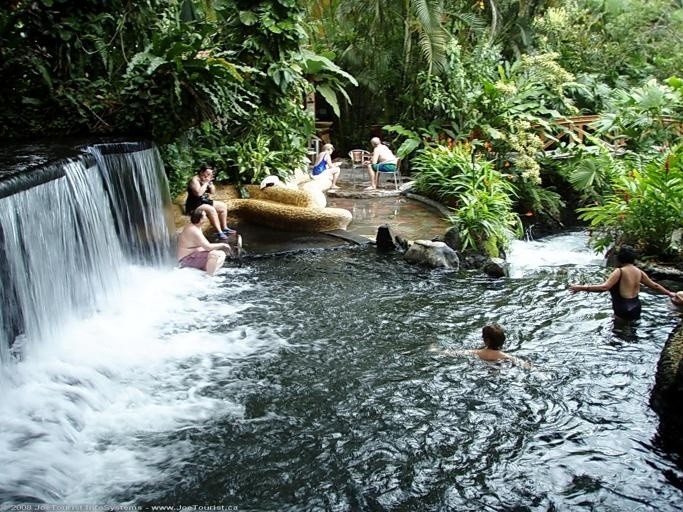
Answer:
[132,183,352,237]
[279,163,332,208]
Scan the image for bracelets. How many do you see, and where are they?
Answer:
[585,285,590,294]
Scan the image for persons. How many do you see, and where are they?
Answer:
[185,164,237,240]
[568,244,679,321]
[176,208,232,283]
[425,323,550,380]
[361,137,397,190]
[310,143,342,189]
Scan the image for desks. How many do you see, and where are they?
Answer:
[361,152,383,189]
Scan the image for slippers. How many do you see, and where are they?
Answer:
[222,227,236,235]
[216,232,227,240]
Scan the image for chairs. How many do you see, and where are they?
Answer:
[375,158,401,191]
[348,149,372,188]
